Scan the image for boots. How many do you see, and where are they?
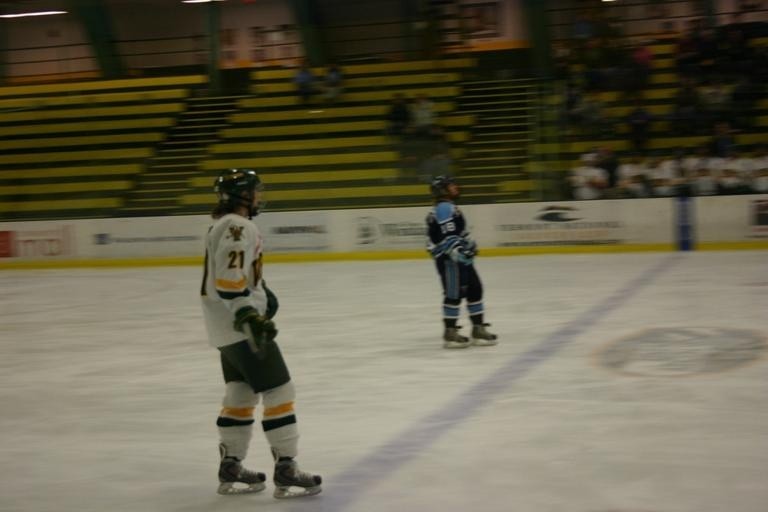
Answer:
[471,325,497,340]
[270,446,321,486]
[218,442,266,483]
[442,327,468,343]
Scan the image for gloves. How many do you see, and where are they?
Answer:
[449,244,476,266]
[233,310,277,343]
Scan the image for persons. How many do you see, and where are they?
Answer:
[321,64,344,95]
[422,177,500,349]
[293,65,317,103]
[552,19,768,198]
[385,93,454,175]
[200,168,325,500]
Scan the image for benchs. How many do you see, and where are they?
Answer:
[1,75,206,220]
[500,46,764,197]
[179,56,478,205]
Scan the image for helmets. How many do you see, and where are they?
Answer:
[430,174,457,199]
[214,168,259,209]
